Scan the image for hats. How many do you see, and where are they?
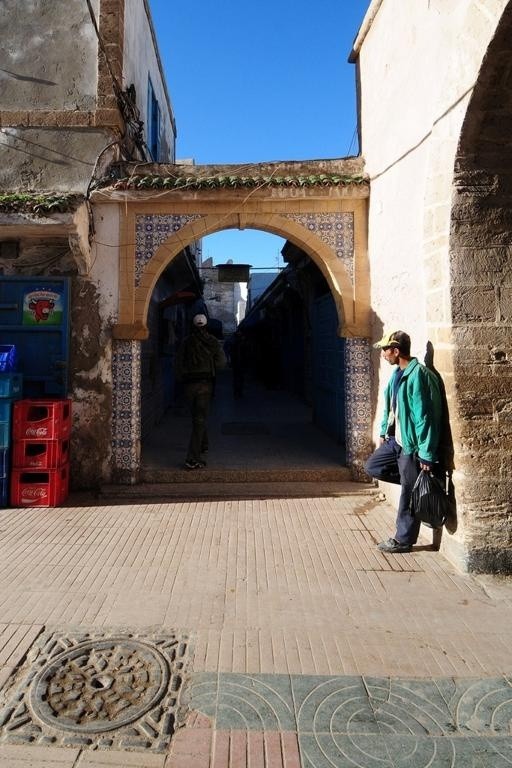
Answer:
[372,330,410,349]
[193,313,207,327]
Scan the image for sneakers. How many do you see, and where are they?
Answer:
[376,538,413,552]
[183,449,209,469]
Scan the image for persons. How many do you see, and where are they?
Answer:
[177,312,228,469]
[364,330,443,552]
